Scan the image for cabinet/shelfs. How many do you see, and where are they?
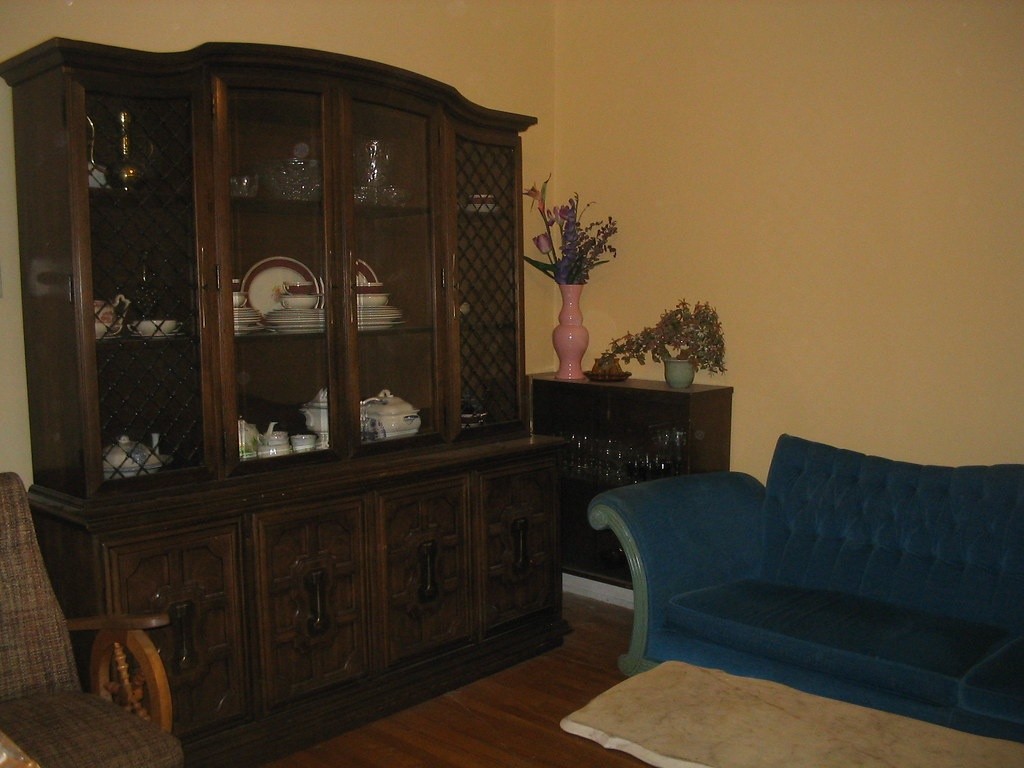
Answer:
[532,372,734,590]
[1,34,575,768]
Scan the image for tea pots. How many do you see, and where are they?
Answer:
[88,109,156,193]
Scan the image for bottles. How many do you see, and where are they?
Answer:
[276,157,321,202]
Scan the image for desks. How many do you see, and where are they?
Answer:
[559,659,1023,768]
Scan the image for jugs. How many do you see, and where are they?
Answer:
[92,294,129,339]
[237,414,277,460]
[354,138,393,207]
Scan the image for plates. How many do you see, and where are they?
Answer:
[460,194,503,207]
[353,258,404,332]
[131,332,186,339]
[232,255,328,334]
[463,208,502,212]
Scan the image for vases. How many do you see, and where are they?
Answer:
[552,285,590,379]
[664,359,696,388]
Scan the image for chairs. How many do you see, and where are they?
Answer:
[0,471,185,768]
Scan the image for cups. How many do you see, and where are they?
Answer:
[553,425,687,483]
[230,173,259,198]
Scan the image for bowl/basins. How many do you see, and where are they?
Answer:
[360,389,422,440]
[257,430,318,458]
[279,295,319,309]
[379,186,417,206]
[232,291,249,307]
[460,413,488,428]
[356,293,391,306]
[131,318,178,338]
[101,434,172,479]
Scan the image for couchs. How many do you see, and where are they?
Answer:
[587,434,1024,744]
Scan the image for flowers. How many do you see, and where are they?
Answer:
[522,173,616,286]
[610,298,728,375]
